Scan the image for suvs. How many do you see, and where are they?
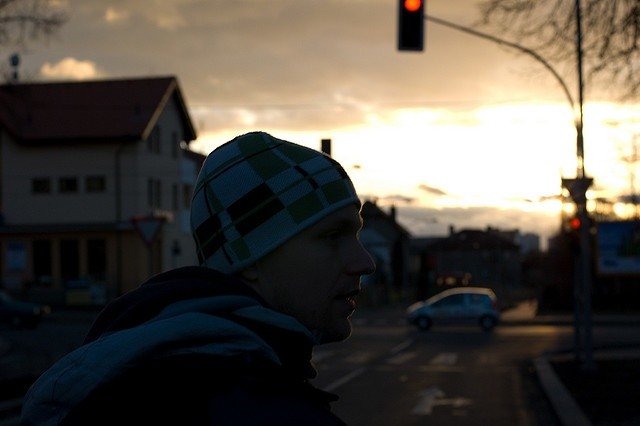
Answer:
[406,287,499,331]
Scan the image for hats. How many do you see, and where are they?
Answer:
[190,131,361,275]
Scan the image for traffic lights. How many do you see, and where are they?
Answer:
[397,0,425,52]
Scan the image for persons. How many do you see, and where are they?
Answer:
[20,131,377,426]
